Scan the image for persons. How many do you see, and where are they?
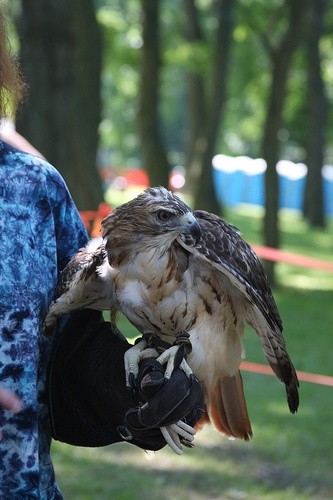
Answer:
[0,3,205,500]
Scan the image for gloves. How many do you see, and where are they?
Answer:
[115,357,205,451]
